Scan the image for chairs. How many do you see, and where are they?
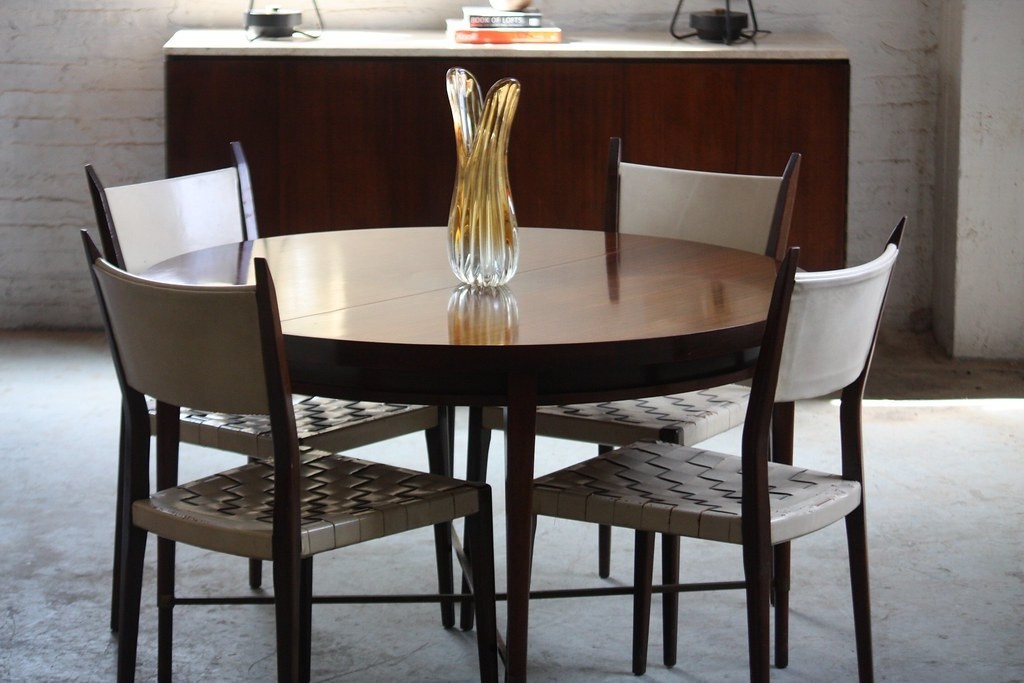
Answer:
[82,226,501,682]
[85,142,457,638]
[458,138,803,605]
[531,217,907,682]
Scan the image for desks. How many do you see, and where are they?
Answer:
[111,225,806,668]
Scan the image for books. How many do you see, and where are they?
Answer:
[446,6,562,44]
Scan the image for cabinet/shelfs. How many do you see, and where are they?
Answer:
[163,56,848,272]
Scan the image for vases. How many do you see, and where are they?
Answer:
[447,282,518,344]
[446,66,522,289]
